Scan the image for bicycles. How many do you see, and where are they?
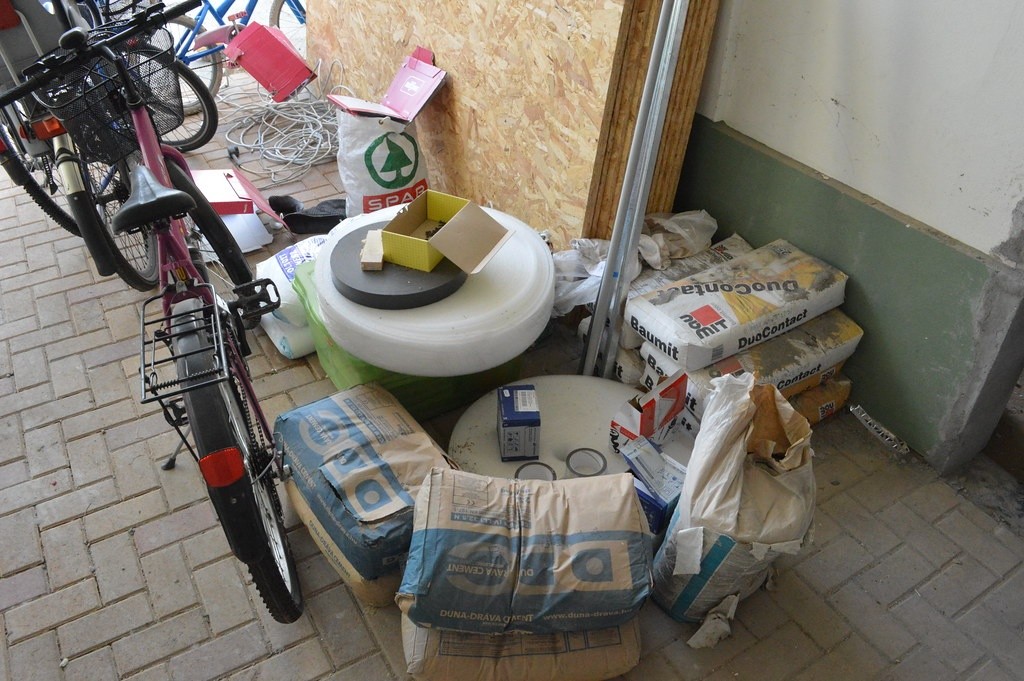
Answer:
[0,0,317,623]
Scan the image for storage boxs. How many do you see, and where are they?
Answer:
[609,371,688,457]
[188,170,296,265]
[620,437,688,535]
[497,385,542,461]
[380,190,515,275]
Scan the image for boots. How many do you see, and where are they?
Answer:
[283,199,346,234]
[269,195,304,215]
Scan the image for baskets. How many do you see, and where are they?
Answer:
[25,19,185,167]
[99,0,163,47]
